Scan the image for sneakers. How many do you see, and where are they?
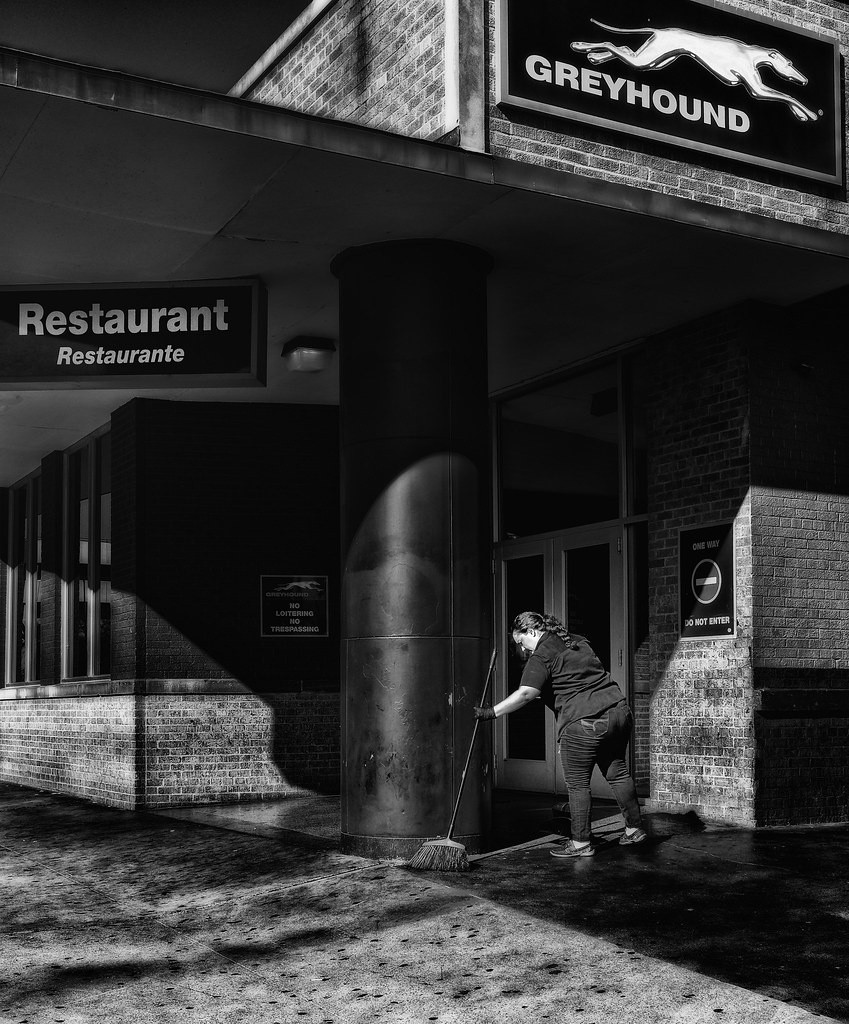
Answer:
[550,837,594,857]
[619,828,647,844]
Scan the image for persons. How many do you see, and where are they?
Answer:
[473,612,646,857]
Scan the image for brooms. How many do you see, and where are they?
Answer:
[405,648,499,872]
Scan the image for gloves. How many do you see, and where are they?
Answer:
[473,706,497,721]
